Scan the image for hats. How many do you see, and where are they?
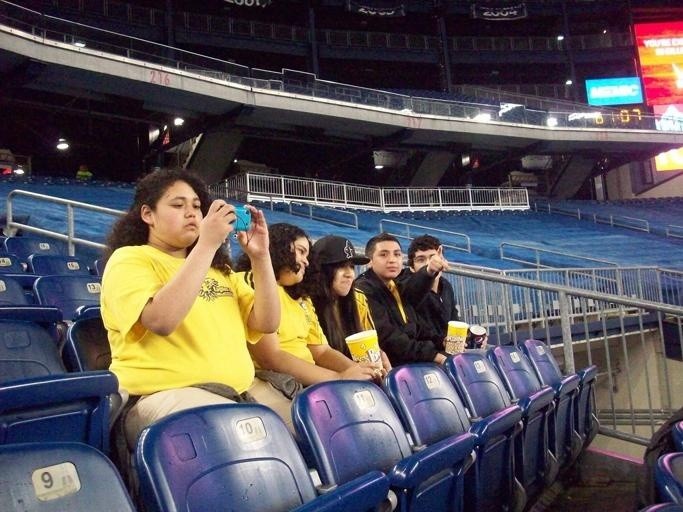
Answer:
[312,236,370,265]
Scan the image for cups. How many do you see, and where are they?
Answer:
[470,325,487,350]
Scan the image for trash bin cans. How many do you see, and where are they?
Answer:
[664,316,683,358]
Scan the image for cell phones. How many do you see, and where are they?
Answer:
[229,207,252,231]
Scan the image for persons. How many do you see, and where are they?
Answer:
[408,234,496,353]
[356,234,467,368]
[99,166,281,451]
[75,164,93,180]
[311,233,390,373]
[2,162,24,176]
[235,221,385,440]
